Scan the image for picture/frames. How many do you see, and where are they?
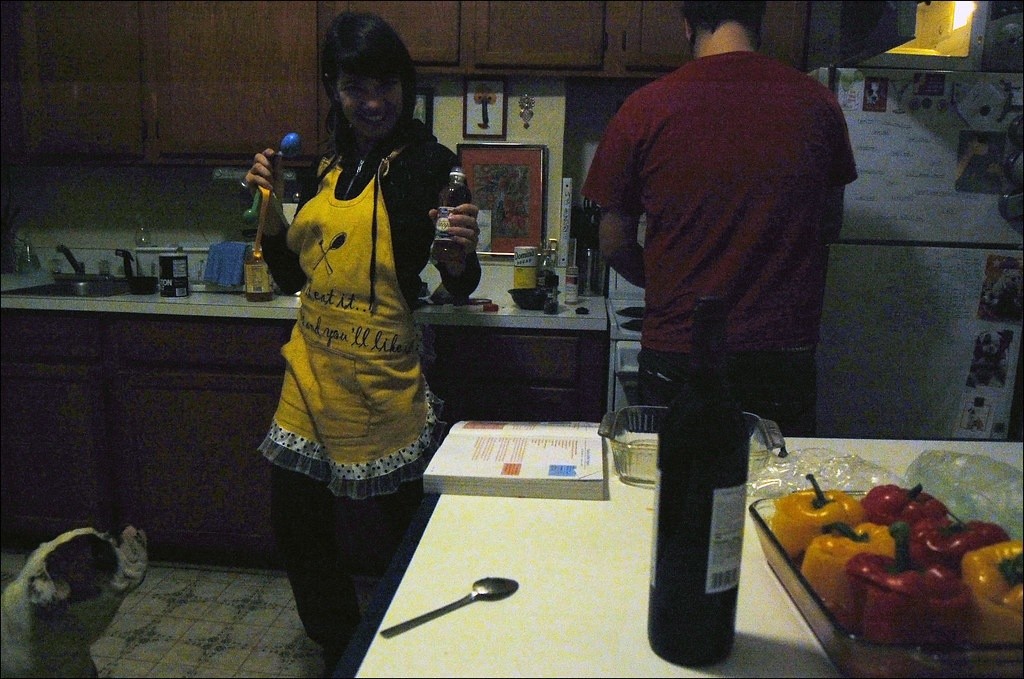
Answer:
[412,86,434,129]
[462,76,508,138]
[456,142,548,258]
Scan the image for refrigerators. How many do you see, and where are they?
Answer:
[792,66,1024,445]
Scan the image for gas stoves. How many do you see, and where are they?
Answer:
[604,210,649,340]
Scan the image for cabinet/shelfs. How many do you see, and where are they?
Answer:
[334,1,468,76]
[0,311,116,555]
[436,326,609,437]
[467,0,812,80]
[1,1,336,168]
[102,313,283,569]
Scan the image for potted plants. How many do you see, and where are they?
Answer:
[1,191,23,274]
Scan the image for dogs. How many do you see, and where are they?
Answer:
[0,522,150,679]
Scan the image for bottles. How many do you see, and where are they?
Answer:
[431,167,469,265]
[646,298,753,667]
[565,265,580,305]
[537,249,555,290]
[243,246,275,302]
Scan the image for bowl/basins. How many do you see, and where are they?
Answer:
[508,287,561,310]
[597,406,784,489]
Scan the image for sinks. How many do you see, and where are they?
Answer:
[3,277,160,296]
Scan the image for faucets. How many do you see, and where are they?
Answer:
[115,249,137,276]
[55,243,86,275]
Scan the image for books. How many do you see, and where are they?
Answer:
[423,421,605,501]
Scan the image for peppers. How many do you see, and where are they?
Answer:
[771,473,1023,679]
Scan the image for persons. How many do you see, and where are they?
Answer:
[245,9,482,678]
[579,0,858,438]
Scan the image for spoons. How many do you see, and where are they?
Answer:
[242,188,265,225]
[270,133,301,163]
[380,578,518,638]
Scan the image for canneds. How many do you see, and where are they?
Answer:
[157,253,190,296]
[244,249,274,302]
[512,245,538,289]
